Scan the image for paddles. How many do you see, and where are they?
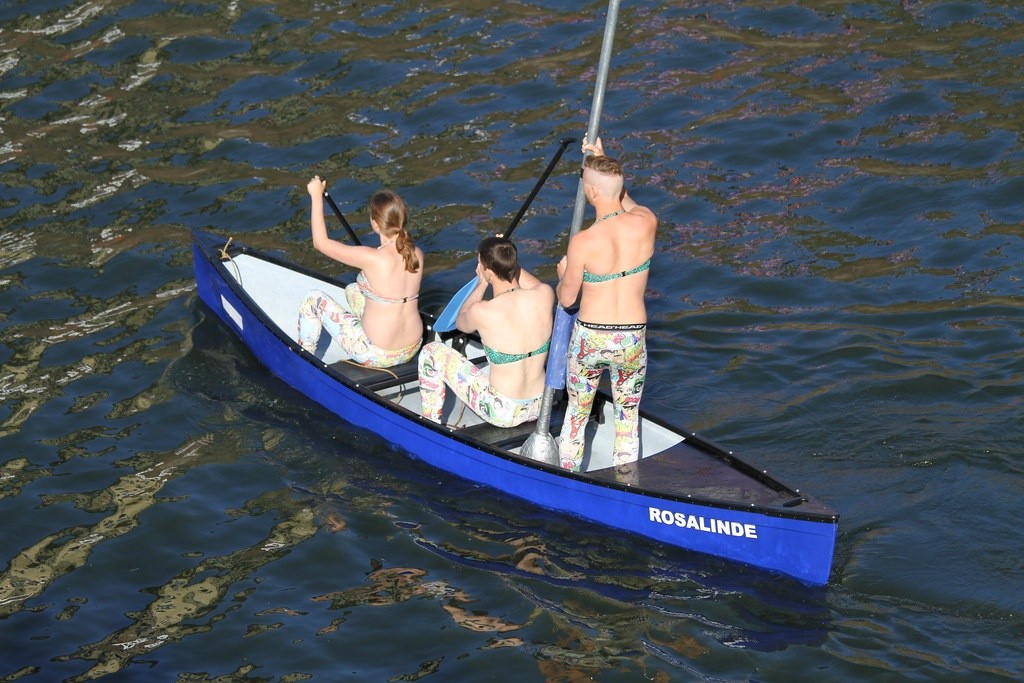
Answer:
[322,188,364,249]
[432,138,576,332]
[521,0,620,464]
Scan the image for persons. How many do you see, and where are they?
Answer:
[417,234,555,428]
[556,131,658,471]
[297,175,424,369]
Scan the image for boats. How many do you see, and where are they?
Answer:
[188,228,841,588]
